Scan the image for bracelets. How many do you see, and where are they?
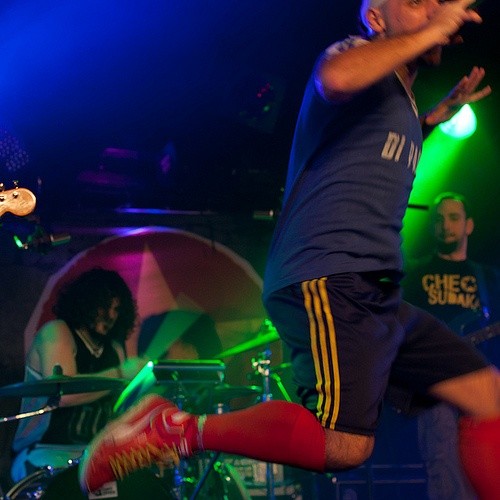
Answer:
[115,365,124,379]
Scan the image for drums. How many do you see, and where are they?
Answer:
[5,467,177,500]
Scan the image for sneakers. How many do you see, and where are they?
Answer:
[78,393,193,494]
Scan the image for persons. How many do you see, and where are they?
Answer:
[77,0,500,500]
[402,191,500,500]
[11,267,138,483]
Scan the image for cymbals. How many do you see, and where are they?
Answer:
[0,375,128,398]
[220,328,281,358]
[191,384,259,413]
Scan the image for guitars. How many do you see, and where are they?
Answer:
[0,181,38,217]
